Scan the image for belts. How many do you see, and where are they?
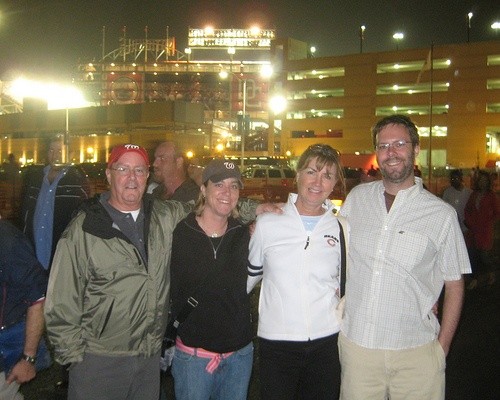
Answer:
[176,336,235,375]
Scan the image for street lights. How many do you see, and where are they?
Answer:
[184,48,192,101]
[491,21,500,40]
[359,24,366,53]
[218,66,273,172]
[392,32,404,50]
[464,8,473,41]
[227,47,235,72]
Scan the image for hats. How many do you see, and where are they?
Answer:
[107,143,151,165]
[202,160,244,190]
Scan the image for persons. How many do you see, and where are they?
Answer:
[42,145,289,400]
[167,158,259,400]
[247,143,439,400]
[367,163,500,289]
[0,132,201,400]
[249,114,471,400]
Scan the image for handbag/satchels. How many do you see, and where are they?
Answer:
[0,319,53,381]
[161,321,178,372]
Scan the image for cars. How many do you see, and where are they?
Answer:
[0,157,500,204]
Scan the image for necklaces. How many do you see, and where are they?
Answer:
[197,215,228,237]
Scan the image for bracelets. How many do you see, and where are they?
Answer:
[22,353,37,363]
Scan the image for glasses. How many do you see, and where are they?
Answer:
[376,139,413,153]
[308,143,342,162]
[109,165,148,179]
